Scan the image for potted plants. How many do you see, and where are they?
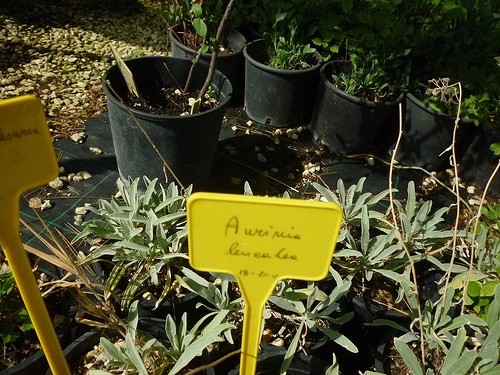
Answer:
[102,0,500,199]
[0,175,500,375]
